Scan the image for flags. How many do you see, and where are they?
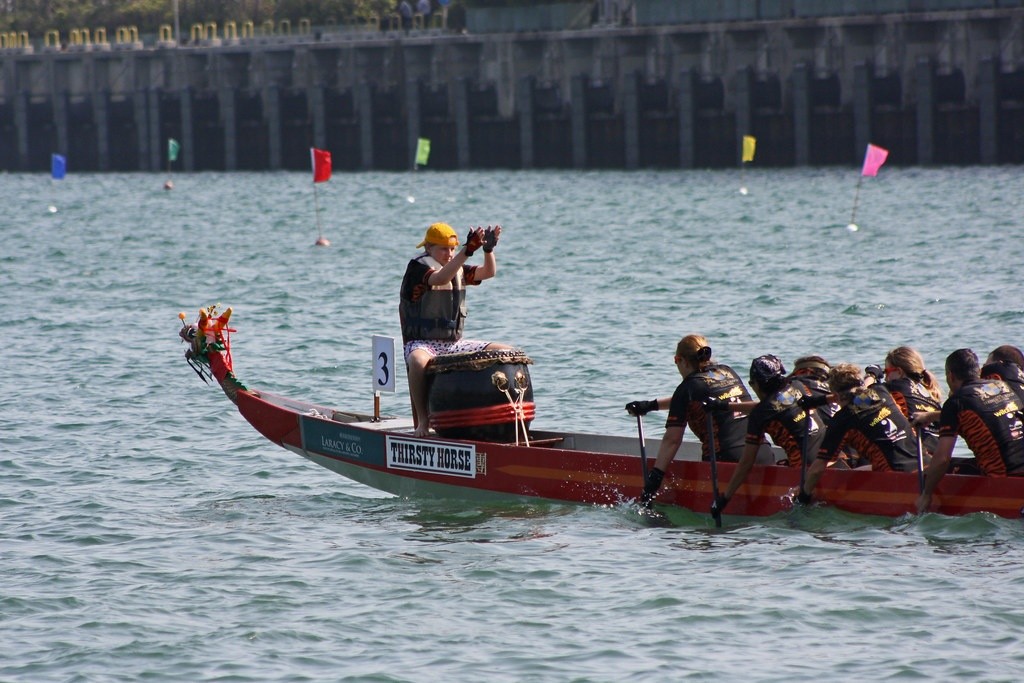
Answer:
[861,144,890,177]
[309,146,332,183]
[741,136,756,161]
[414,137,431,165]
[167,138,180,161]
[50,153,68,180]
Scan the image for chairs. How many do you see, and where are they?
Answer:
[397,303,434,428]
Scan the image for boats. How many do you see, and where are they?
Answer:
[175,302,1024,517]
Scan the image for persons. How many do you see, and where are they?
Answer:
[626,333,1024,520]
[398,223,502,439]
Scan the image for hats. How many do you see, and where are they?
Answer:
[749,354,786,382]
[786,362,830,379]
[415,223,459,249]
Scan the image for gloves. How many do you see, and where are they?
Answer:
[644,467,665,498]
[700,396,730,414]
[864,363,884,385]
[711,493,731,519]
[798,394,829,411]
[794,490,813,507]
[625,399,659,417]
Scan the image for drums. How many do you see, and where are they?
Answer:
[425,349,536,444]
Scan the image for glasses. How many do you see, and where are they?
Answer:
[884,367,899,375]
[748,379,757,387]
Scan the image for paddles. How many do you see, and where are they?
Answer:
[908,415,926,514]
[699,400,722,527]
[877,370,886,382]
[626,403,653,510]
[791,399,811,528]
